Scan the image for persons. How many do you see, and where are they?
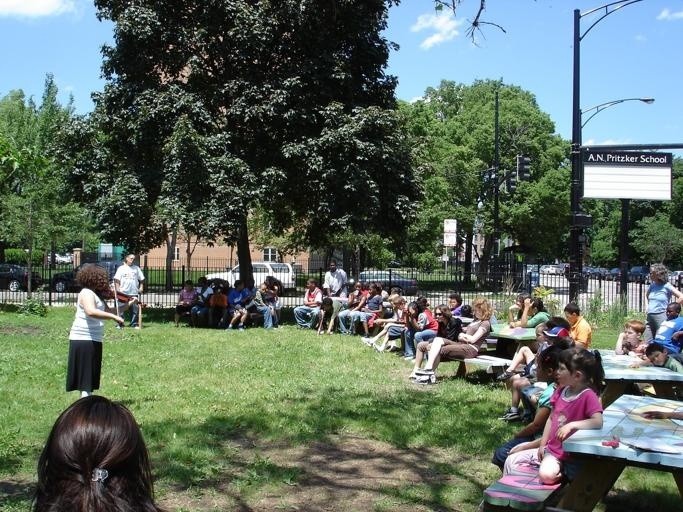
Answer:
[28,394,160,512]
[293,279,493,385]
[508,294,593,349]
[641,410,682,421]
[110,252,145,328]
[174,277,283,332]
[322,260,348,298]
[504,346,605,486]
[66,261,123,397]
[614,263,682,375]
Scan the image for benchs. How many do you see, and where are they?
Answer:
[180,311,264,328]
[483,466,560,511]
[450,354,511,377]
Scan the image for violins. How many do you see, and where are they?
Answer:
[104,289,146,306]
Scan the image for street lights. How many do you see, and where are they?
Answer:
[565,0,655,309]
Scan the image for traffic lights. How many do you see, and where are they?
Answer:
[518,153,531,182]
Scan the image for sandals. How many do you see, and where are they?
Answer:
[496,371,513,380]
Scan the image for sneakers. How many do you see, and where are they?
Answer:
[497,407,533,423]
[408,367,438,386]
[361,336,384,353]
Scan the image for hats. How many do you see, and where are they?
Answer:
[542,326,569,338]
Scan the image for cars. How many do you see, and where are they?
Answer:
[47,254,71,264]
[347,271,417,295]
[525,261,683,288]
[0,264,41,292]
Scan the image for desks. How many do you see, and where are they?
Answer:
[321,289,497,344]
[556,393,683,510]
[585,349,683,409]
[490,323,537,359]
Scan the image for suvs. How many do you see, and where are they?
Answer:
[53,260,122,293]
[205,261,294,297]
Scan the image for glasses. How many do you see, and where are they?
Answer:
[435,313,442,316]
